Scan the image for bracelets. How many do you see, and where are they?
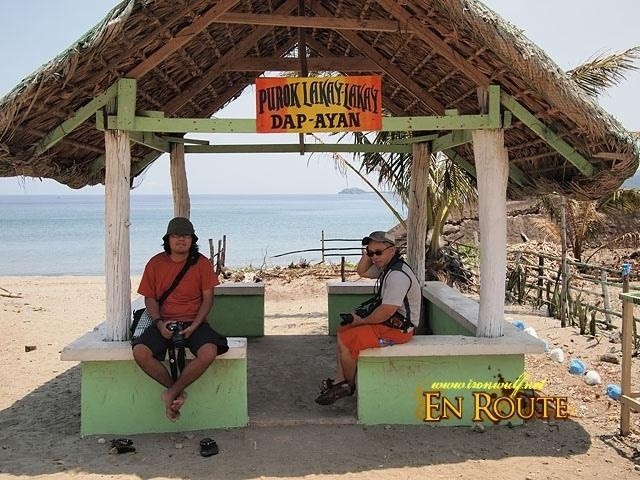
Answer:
[153,318,164,324]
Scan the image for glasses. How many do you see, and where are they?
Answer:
[366,246,393,257]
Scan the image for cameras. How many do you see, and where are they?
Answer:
[167,322,188,347]
[339,305,370,328]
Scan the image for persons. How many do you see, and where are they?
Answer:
[312,230,422,408]
[131,216,230,423]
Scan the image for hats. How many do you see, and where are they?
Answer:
[362,231,395,245]
[167,217,194,235]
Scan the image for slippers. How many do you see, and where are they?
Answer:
[200,438,218,456]
[112,438,135,452]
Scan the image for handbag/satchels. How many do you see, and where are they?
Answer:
[131,308,153,340]
[355,299,412,330]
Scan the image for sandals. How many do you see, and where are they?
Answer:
[314,378,355,405]
